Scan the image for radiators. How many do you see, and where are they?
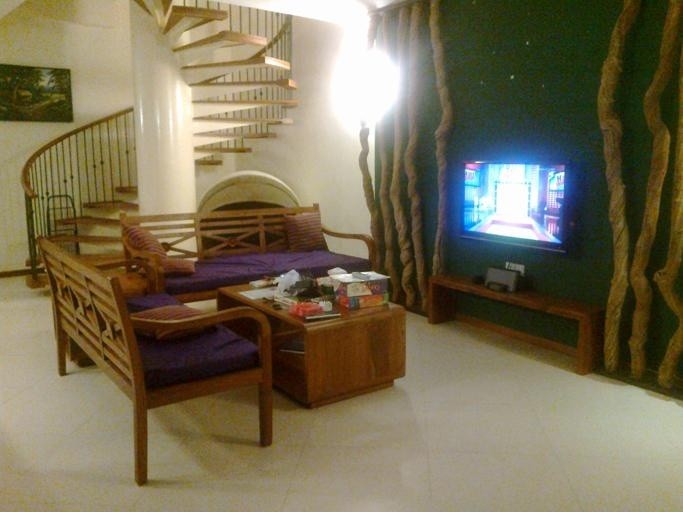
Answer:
[304,313,341,320]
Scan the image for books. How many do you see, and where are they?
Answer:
[330,271,390,309]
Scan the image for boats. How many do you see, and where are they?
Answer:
[484,267,520,293]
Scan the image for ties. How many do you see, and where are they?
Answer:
[458,158,570,256]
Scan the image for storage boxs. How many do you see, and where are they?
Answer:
[330,271,390,309]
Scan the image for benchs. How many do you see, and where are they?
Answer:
[36,204,377,312]
[40,238,274,485]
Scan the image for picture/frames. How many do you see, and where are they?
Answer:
[427,275,606,377]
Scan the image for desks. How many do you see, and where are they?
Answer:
[427,275,606,377]
[218,282,405,410]
